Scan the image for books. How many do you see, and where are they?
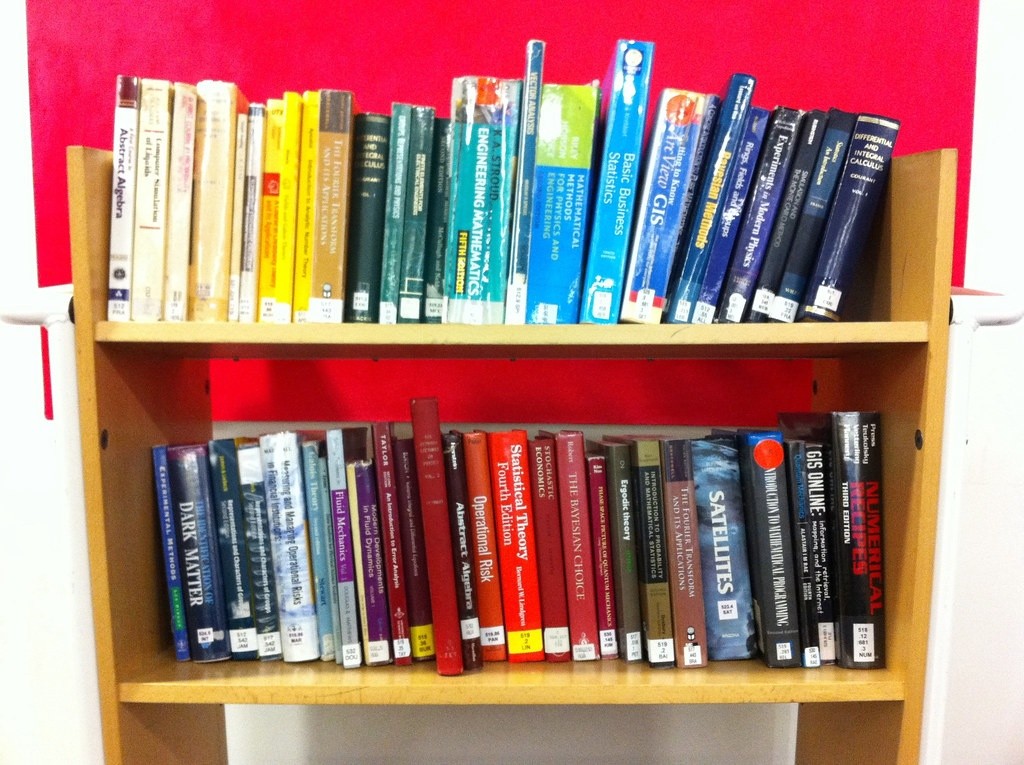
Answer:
[104,37,900,326]
[151,394,888,676]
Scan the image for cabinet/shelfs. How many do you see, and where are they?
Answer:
[66,144,959,765]
